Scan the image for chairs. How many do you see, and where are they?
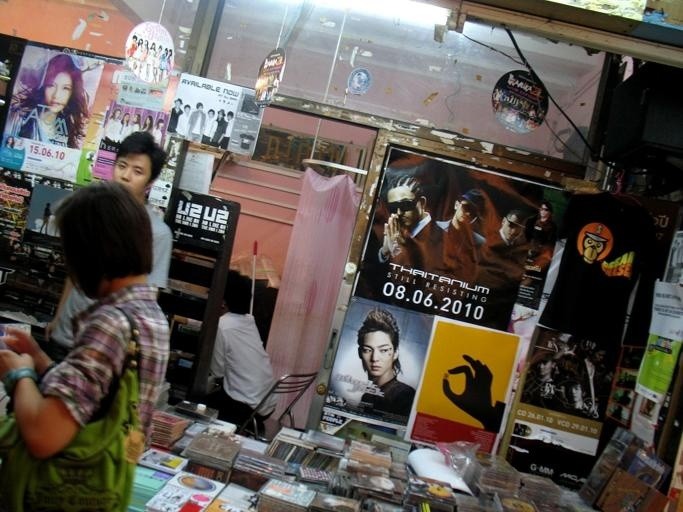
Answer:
[237,371,319,443]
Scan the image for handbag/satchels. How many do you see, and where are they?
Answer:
[2,306,143,511]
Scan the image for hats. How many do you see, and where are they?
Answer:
[462,189,485,216]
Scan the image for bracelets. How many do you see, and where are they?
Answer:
[37,361,59,384]
[3,368,38,396]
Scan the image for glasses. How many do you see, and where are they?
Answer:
[387,197,418,214]
[462,204,475,216]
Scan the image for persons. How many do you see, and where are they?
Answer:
[357,309,416,424]
[0,180,170,512]
[5,136,14,149]
[10,54,90,149]
[166,98,234,149]
[40,203,51,235]
[202,270,277,433]
[377,173,556,304]
[526,333,607,419]
[102,108,164,148]
[127,34,172,83]
[443,355,505,432]
[42,132,174,363]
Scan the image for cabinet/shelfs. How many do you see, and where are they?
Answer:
[157,189,240,406]
